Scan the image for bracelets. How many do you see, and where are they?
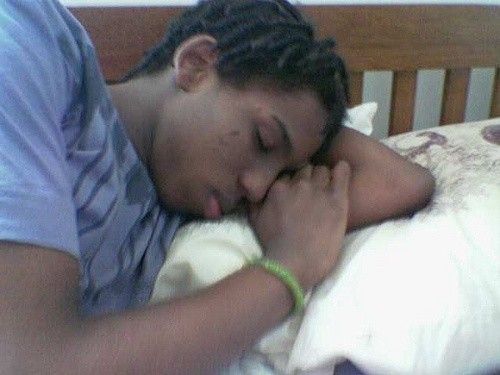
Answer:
[243,255,307,318]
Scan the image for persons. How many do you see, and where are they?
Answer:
[0,0,438,375]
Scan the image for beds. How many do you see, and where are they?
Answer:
[66,3,500,375]
